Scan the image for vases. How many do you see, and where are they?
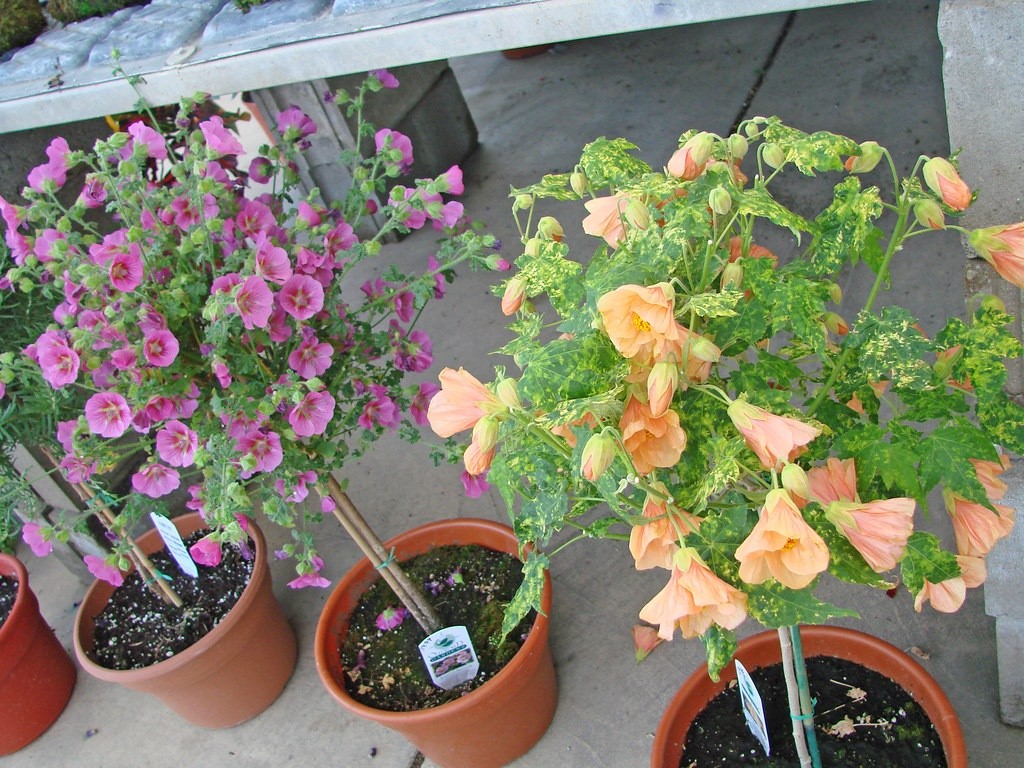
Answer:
[650,625,969,768]
[312,517,559,768]
[73,509,299,731]
[0,552,77,756]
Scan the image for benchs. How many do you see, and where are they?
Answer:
[0,0,868,250]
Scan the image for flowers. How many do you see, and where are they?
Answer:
[0,44,1024,768]
